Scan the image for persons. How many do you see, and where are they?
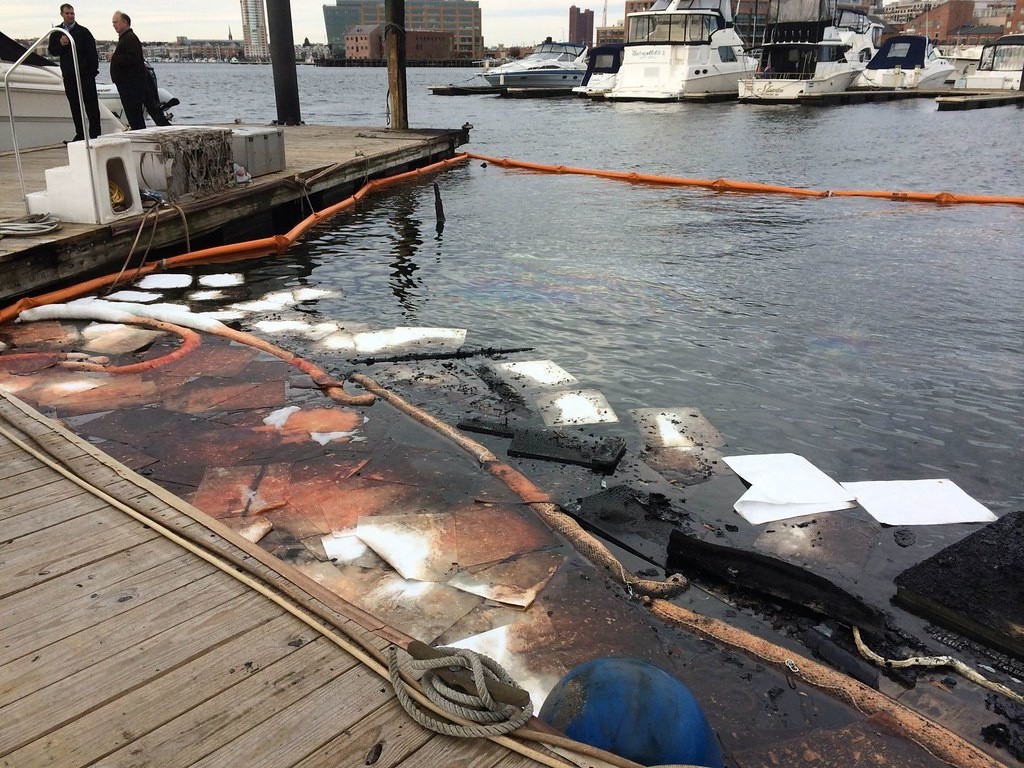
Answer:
[105,11,149,130]
[48,4,102,145]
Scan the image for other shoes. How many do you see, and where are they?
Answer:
[63,140,72,145]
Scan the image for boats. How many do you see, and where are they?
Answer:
[850,35,955,89]
[94,82,179,124]
[739,0,893,103]
[945,34,1024,91]
[604,0,758,102]
[0,31,126,150]
[572,44,623,92]
[426,82,508,95]
[476,38,590,87]
